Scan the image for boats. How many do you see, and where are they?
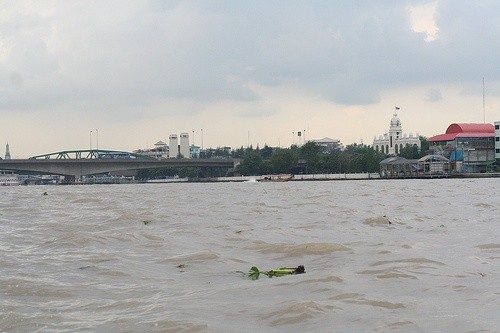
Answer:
[254,174,294,182]
